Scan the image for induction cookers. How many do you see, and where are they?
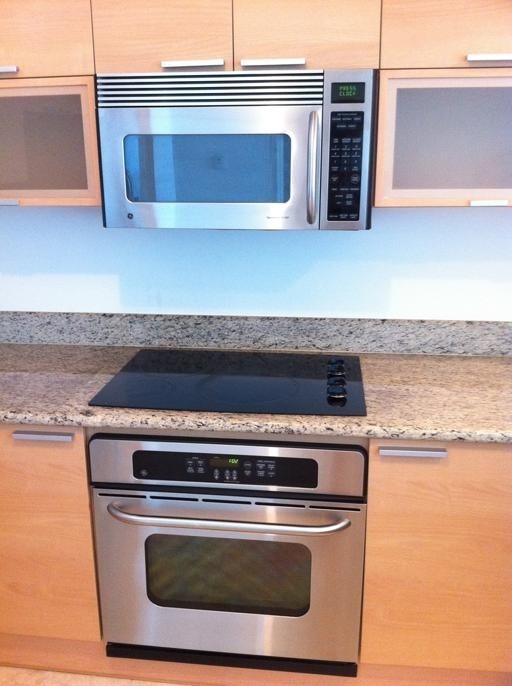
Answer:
[88,348,367,417]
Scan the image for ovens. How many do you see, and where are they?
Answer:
[89,431,368,677]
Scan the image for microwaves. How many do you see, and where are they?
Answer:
[96,67,377,232]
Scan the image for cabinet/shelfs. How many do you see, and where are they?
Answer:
[0,0,103,209]
[91,0,382,81]
[373,0,512,209]
[2,424,104,653]
[359,439,512,674]
